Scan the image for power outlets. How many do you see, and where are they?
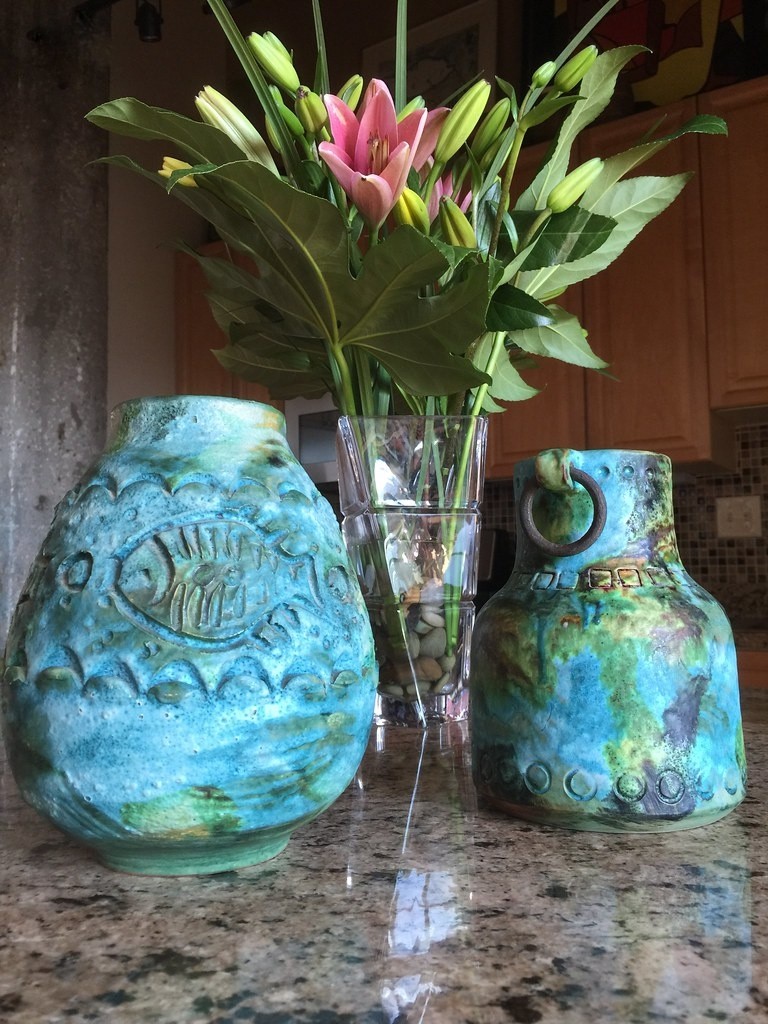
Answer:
[712,493,764,540]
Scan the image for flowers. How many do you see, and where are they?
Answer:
[84,0,732,418]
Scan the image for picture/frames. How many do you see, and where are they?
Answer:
[356,0,528,122]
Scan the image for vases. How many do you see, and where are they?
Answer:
[338,412,497,736]
[0,391,382,883]
[463,451,749,840]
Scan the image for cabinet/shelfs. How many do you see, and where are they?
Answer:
[163,71,768,484]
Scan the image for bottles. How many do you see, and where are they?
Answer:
[0,395,380,878]
[469,447,748,833]
